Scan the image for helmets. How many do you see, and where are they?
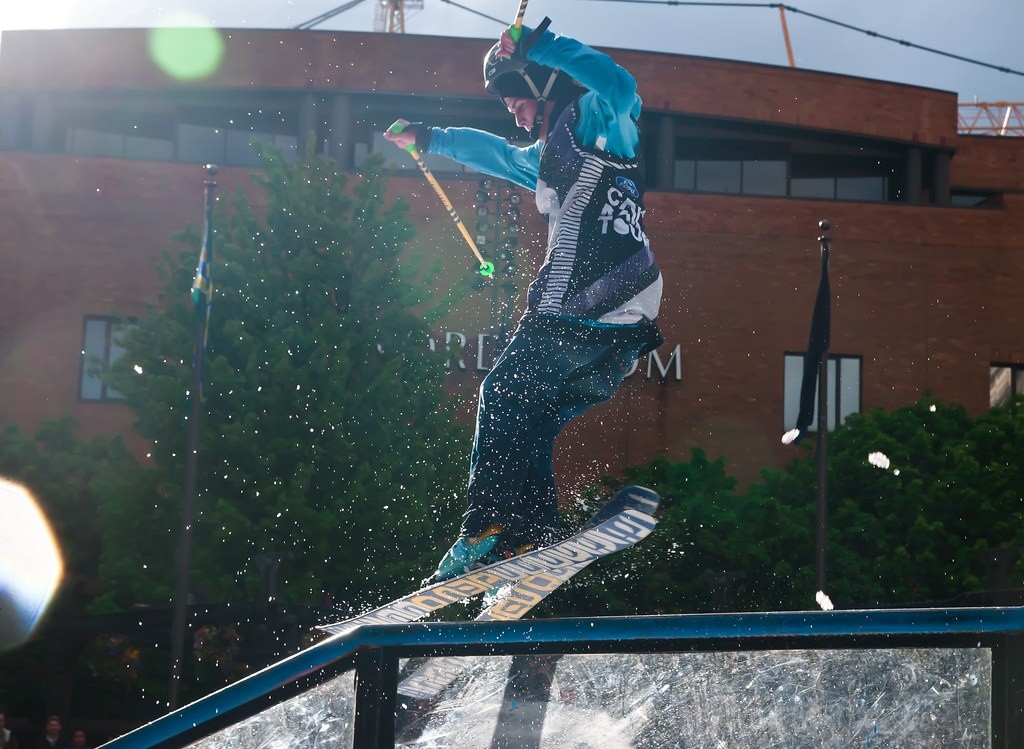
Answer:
[484,40,529,94]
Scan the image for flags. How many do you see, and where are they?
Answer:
[191,209,213,405]
[796,243,830,435]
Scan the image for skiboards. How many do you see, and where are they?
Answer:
[311,482,660,741]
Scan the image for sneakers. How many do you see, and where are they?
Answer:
[434,519,546,584]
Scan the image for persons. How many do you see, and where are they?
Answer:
[383,16,662,590]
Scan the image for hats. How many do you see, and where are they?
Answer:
[497,61,578,106]
